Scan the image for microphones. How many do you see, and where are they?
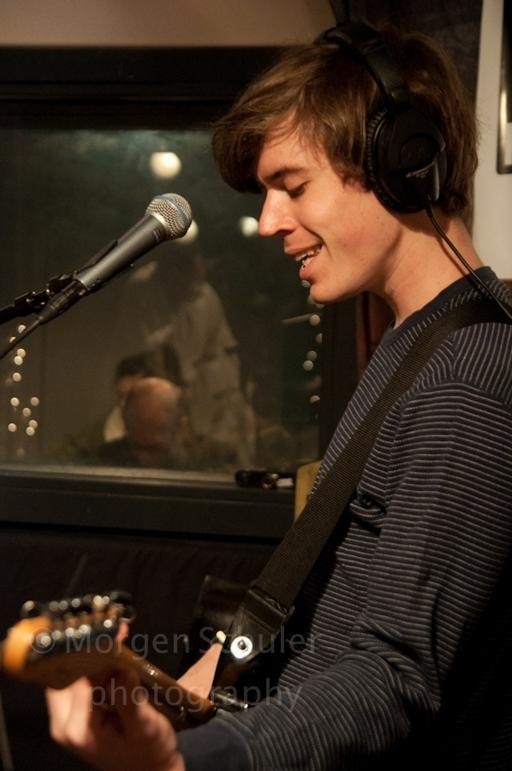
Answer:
[36,192,196,327]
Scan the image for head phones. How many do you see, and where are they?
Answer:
[312,23,457,214]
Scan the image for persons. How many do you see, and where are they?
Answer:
[141,239,257,461]
[118,375,212,471]
[98,339,264,467]
[39,19,510,770]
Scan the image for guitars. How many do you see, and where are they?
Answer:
[2,591,301,730]
